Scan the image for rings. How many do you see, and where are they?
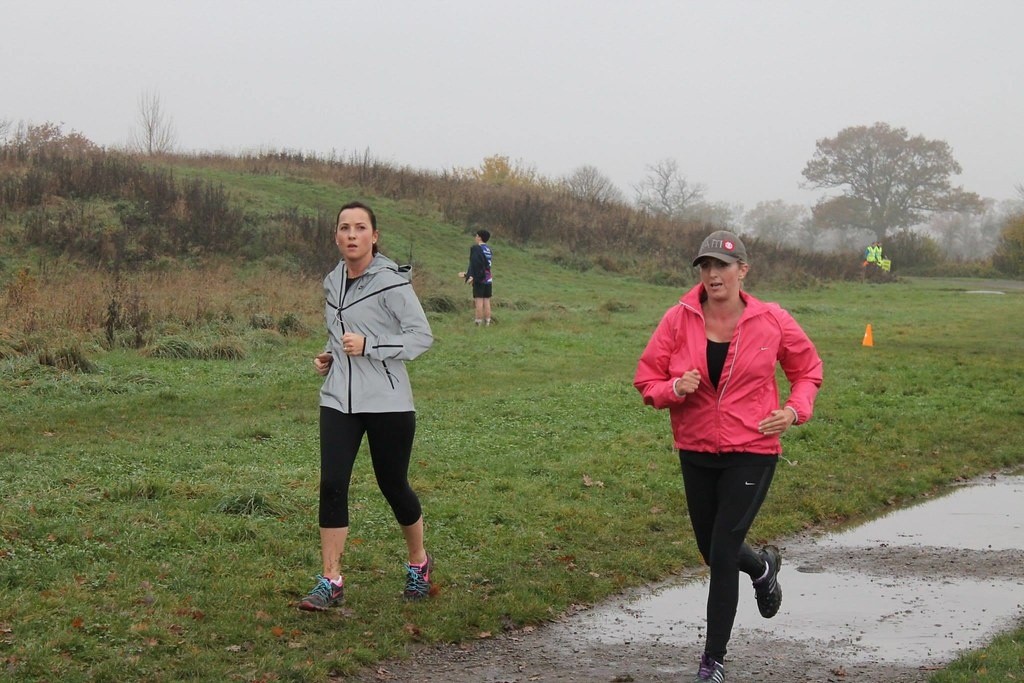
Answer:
[344,347,346,351]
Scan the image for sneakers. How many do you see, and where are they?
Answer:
[751,544,783,618]
[299,575,346,611]
[404,553,431,602]
[693,653,725,683]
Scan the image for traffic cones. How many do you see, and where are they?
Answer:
[861,324,874,348]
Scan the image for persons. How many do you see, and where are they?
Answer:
[299,202,434,611]
[633,230,823,683]
[863,239,886,272]
[458,230,493,327]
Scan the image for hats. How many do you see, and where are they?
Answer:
[693,230,749,267]
